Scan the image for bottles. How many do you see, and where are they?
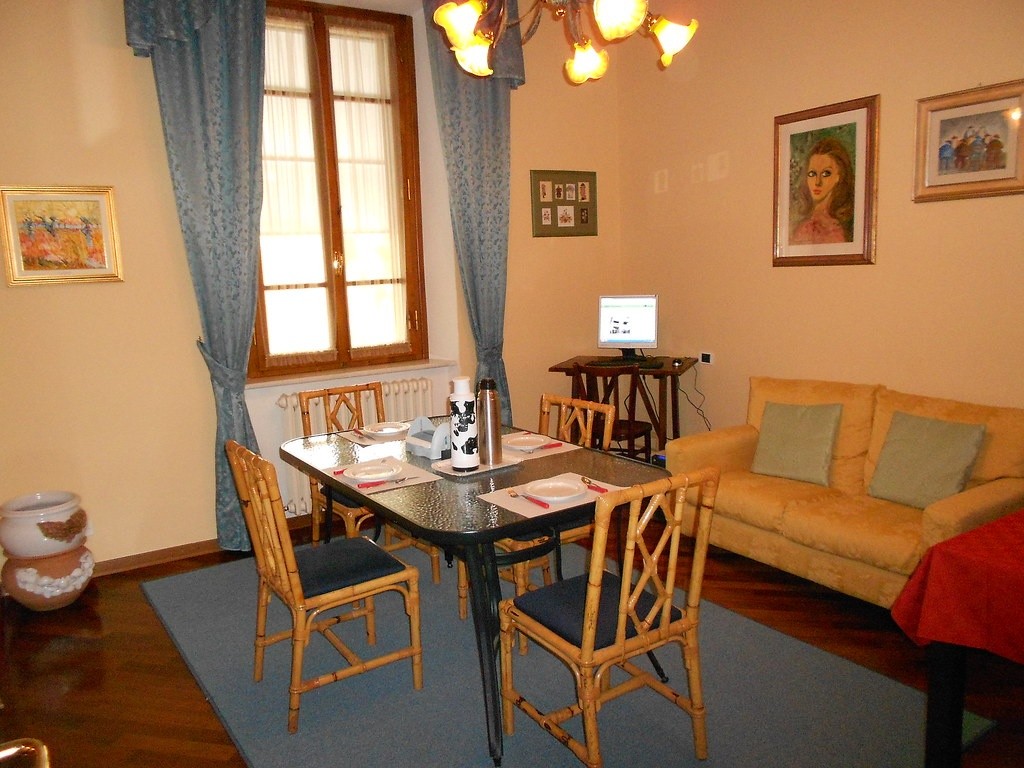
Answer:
[450,377,480,472]
[475,378,502,466]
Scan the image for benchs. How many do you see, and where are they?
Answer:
[660,376,1024,612]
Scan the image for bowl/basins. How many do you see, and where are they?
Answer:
[0,490,96,612]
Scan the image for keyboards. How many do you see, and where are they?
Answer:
[585,360,664,369]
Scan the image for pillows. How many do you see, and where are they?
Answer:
[867,410,987,511]
[750,400,844,488]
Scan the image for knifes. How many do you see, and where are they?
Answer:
[358,477,420,488]
[353,427,375,441]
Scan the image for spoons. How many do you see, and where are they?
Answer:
[521,443,562,453]
[581,476,609,492]
[509,490,549,509]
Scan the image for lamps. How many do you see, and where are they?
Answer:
[433,0,699,87]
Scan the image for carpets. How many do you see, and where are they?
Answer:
[139,525,998,768]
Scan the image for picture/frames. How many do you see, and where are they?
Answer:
[773,93,881,268]
[0,184,125,287]
[914,78,1024,204]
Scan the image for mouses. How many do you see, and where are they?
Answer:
[672,358,683,367]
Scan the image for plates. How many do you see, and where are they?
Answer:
[343,462,402,481]
[364,422,410,436]
[524,479,586,500]
[505,434,552,449]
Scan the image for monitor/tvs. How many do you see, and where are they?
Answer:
[597,294,658,360]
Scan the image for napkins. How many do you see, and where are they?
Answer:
[322,455,444,496]
[336,420,415,447]
[477,471,632,520]
[501,430,584,462]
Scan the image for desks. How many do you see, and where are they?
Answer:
[886,506,1024,768]
[549,354,699,450]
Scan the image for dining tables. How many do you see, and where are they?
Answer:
[278,413,673,768]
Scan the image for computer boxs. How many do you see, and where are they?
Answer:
[651,450,666,468]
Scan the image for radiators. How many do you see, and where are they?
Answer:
[275,377,434,519]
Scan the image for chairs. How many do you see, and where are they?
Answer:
[297,381,442,610]
[499,466,721,768]
[223,439,422,736]
[570,362,653,464]
[459,392,617,658]
